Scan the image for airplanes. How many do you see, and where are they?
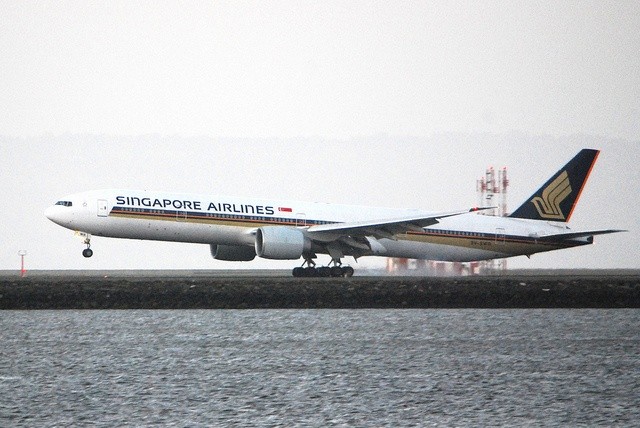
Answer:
[42,148,630,277]
